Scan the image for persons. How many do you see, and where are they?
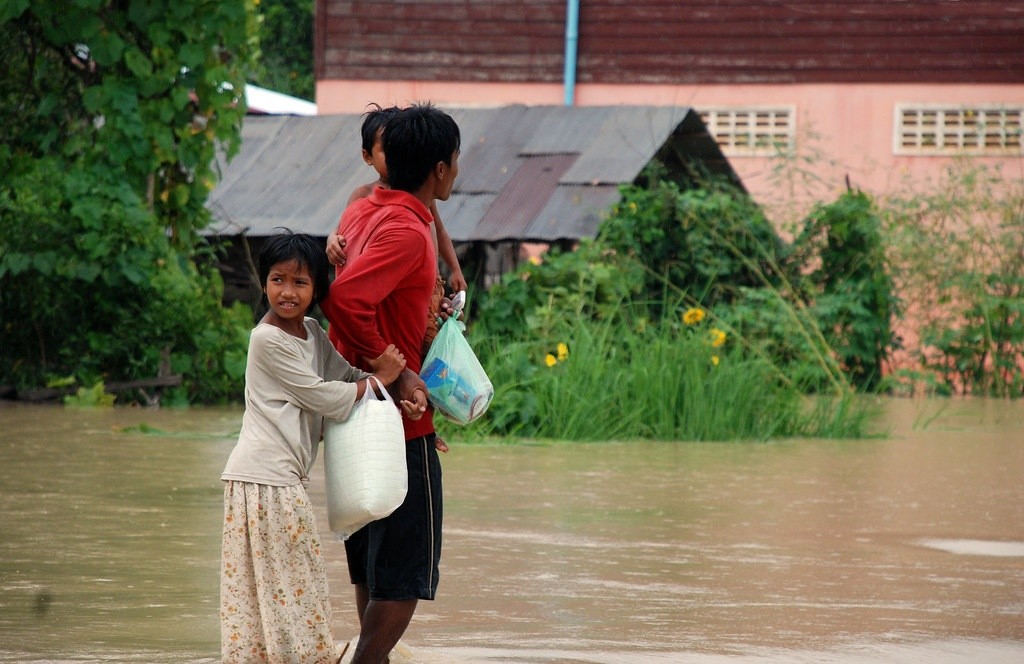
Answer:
[327,102,468,453]
[220,226,406,664]
[325,100,461,664]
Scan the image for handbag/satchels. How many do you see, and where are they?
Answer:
[325,376,408,537]
[421,311,494,426]
